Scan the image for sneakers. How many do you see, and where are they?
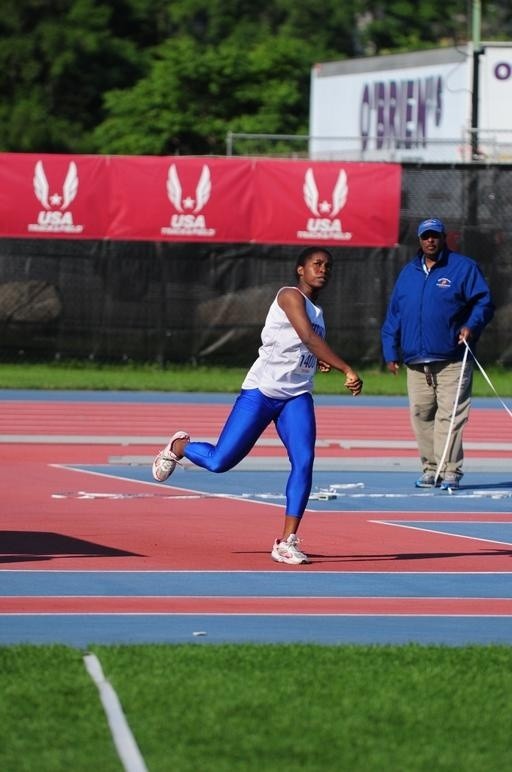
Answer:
[414,469,460,498]
[269,532,311,569]
[149,429,189,483]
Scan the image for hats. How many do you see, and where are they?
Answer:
[417,217,444,240]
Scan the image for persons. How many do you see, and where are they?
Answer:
[151,246,363,567]
[377,216,499,490]
